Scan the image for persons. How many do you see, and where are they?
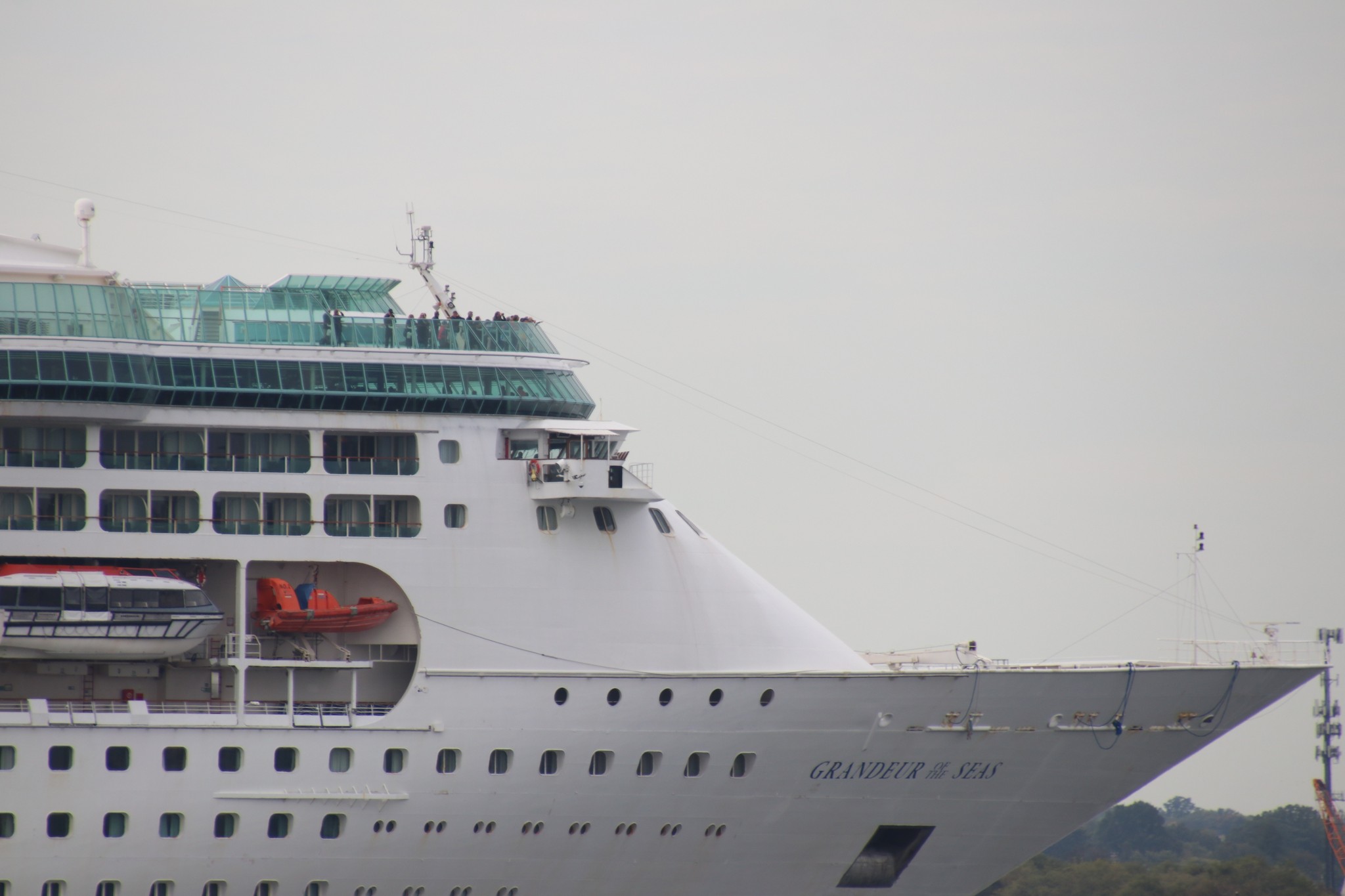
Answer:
[471,390,477,395]
[323,309,343,347]
[442,379,454,394]
[500,386,539,398]
[388,386,397,392]
[534,454,538,458]
[514,451,523,459]
[383,309,396,347]
[403,310,543,352]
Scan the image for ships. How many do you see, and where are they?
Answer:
[0,195,1336,896]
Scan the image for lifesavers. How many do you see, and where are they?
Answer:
[529,460,540,474]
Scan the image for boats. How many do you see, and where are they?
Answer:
[251,574,402,634]
[0,558,222,664]
